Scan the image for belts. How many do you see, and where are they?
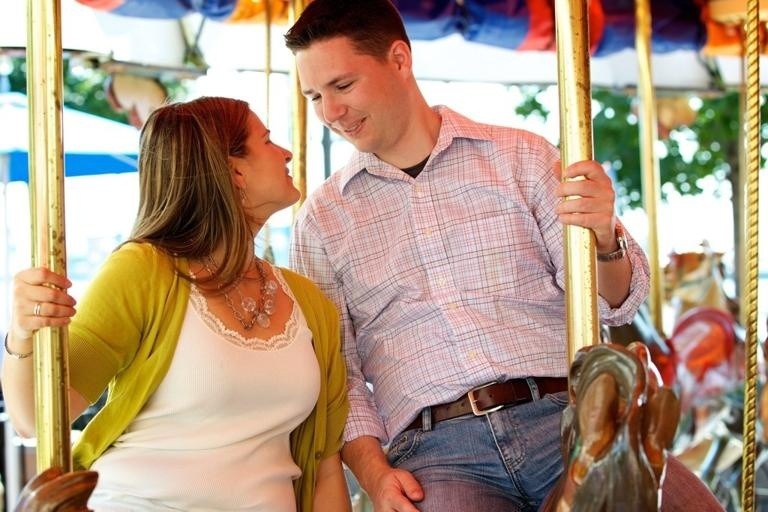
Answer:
[405,372,569,433]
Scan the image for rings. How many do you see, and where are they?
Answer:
[33,301,40,316]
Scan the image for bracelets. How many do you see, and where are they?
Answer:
[4,333,33,360]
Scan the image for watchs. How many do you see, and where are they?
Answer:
[596,222,628,264]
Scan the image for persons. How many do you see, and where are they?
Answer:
[284,1,727,512]
[0,96,352,512]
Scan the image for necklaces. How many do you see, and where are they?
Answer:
[197,247,276,332]
